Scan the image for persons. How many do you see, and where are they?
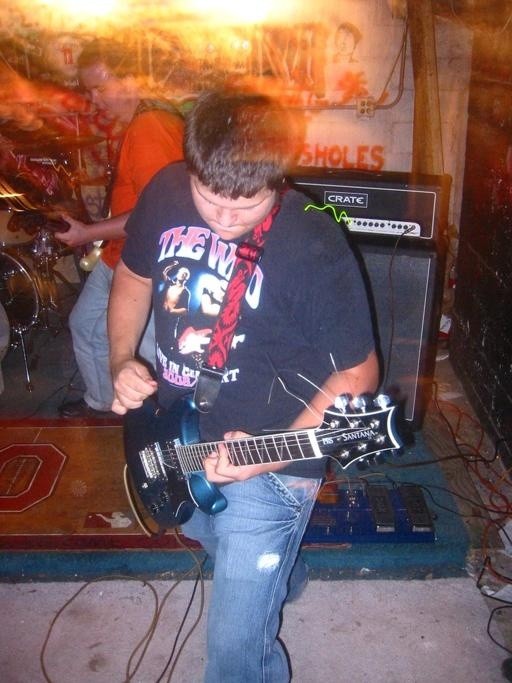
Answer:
[51,35,192,418]
[329,20,365,66]
[107,80,384,683]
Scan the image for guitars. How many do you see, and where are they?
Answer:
[7,205,114,271]
[124,394,406,526]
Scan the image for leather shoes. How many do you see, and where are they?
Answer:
[56,397,115,418]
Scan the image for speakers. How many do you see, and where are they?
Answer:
[356,246,445,431]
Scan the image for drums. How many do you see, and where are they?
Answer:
[0,210,37,246]
[0,250,60,333]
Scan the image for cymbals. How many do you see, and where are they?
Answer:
[12,134,105,158]
